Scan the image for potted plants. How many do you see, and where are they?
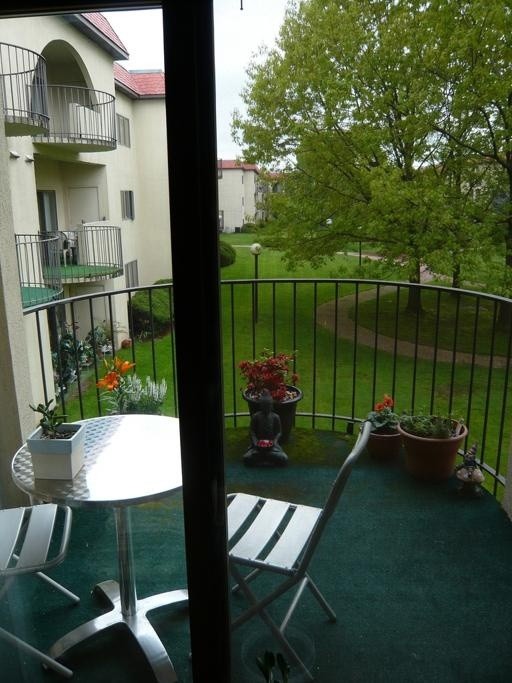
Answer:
[28,400,85,482]
[238,347,303,438]
[397,415,468,480]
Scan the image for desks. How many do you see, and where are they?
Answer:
[9,412,188,683]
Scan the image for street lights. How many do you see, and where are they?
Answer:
[250,243,262,322]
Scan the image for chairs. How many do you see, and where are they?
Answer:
[0,503,81,677]
[44,230,77,267]
[224,419,375,680]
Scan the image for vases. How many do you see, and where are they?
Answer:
[358,427,401,462]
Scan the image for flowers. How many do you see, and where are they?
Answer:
[361,393,400,433]
[95,338,136,414]
